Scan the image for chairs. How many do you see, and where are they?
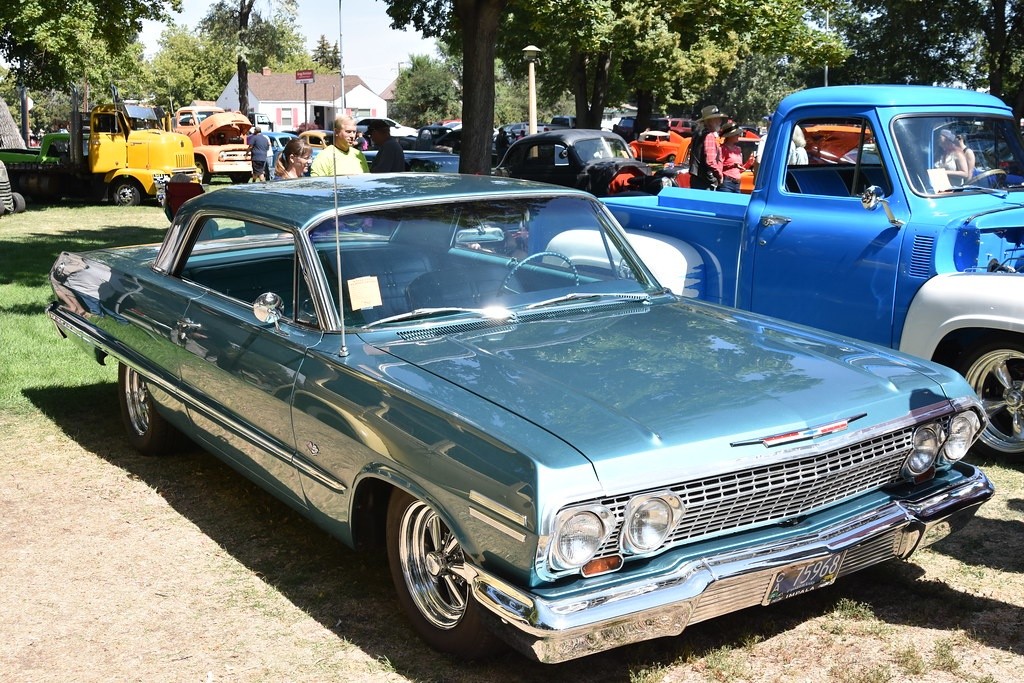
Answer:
[786,167,851,197]
[861,166,891,199]
[405,266,526,314]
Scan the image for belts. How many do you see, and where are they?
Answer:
[723,176,740,184]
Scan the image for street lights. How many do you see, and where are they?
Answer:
[522,44,541,135]
[331,70,345,112]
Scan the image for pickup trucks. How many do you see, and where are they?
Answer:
[524,85,1024,460]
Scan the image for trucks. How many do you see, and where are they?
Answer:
[0,78,254,211]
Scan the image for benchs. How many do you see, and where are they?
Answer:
[182,247,440,329]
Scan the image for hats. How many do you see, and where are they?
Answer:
[940,129,955,142]
[254,127,261,133]
[696,105,729,123]
[364,120,389,135]
[719,122,743,138]
[764,115,774,123]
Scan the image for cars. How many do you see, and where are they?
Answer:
[49,170,996,663]
[263,113,760,197]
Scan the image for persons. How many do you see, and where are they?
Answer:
[365,119,406,173]
[689,104,809,193]
[312,116,370,177]
[496,127,556,168]
[935,127,976,186]
[244,128,269,182]
[271,138,313,181]
